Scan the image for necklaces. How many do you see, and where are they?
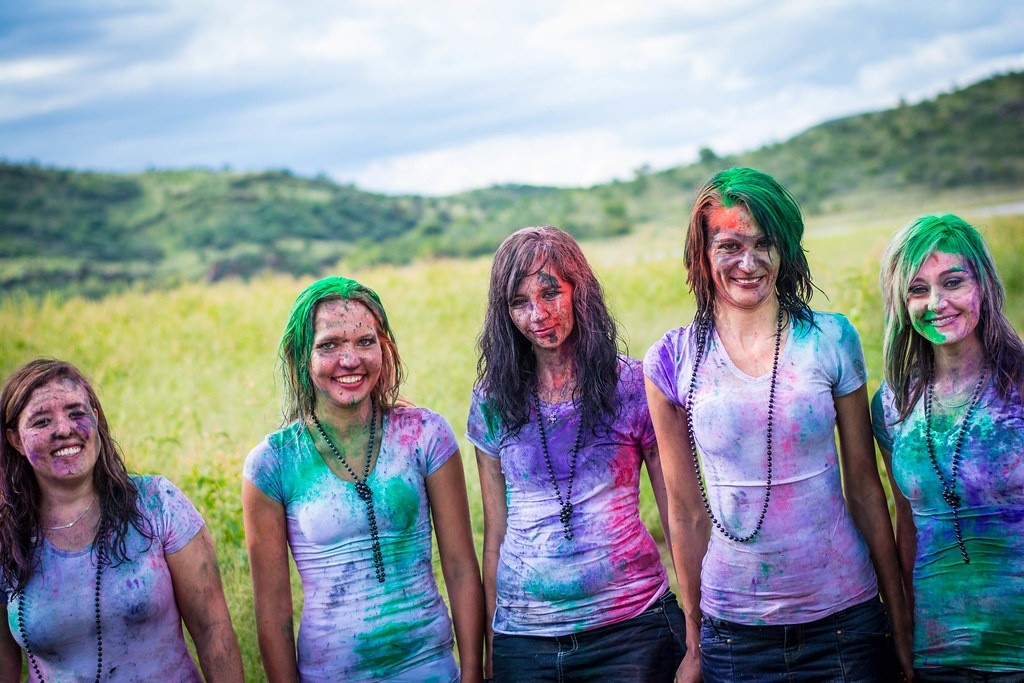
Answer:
[933,395,972,408]
[536,369,573,424]
[42,501,95,530]
[310,397,386,582]
[925,361,987,564]
[532,381,583,541]
[18,516,105,683]
[685,296,783,541]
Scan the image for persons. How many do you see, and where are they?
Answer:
[643,168,914,683]
[871,214,1024,683]
[241,276,484,683]
[466,225,686,683]
[0,359,245,683]
